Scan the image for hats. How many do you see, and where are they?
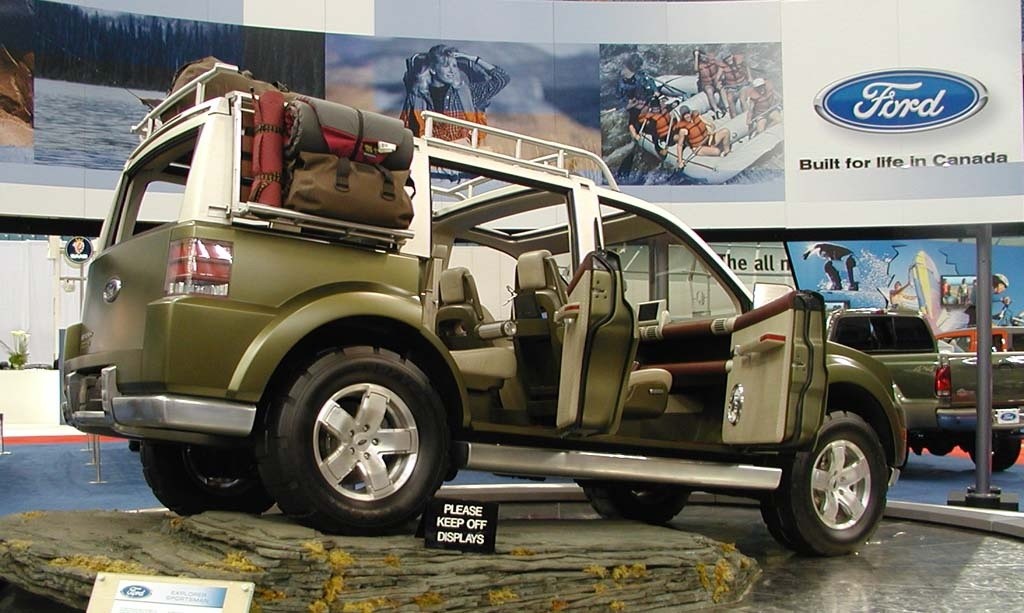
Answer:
[751,77,766,88]
[650,96,659,106]
[679,106,690,116]
[620,69,635,81]
[720,51,730,61]
[994,272,1009,288]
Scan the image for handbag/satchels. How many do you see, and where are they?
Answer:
[287,153,415,228]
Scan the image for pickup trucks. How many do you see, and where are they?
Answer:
[826,303,1024,471]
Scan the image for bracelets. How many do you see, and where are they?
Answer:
[475,56,482,64]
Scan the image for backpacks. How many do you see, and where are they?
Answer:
[403,53,475,124]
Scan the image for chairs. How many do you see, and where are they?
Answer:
[433,249,672,418]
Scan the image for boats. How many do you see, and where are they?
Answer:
[630,73,785,184]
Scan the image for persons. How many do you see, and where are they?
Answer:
[648,96,679,157]
[716,50,752,118]
[677,105,731,169]
[399,44,511,146]
[957,278,969,304]
[943,278,951,304]
[970,280,977,304]
[693,48,724,114]
[803,243,861,291]
[961,273,1010,328]
[747,78,782,134]
[890,281,919,310]
[629,96,651,141]
[618,54,659,108]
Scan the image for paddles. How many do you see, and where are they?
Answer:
[752,103,782,122]
[658,104,676,169]
[617,108,652,175]
[672,135,709,178]
[699,53,768,74]
[644,72,687,94]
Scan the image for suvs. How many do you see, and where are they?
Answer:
[54,55,907,558]
